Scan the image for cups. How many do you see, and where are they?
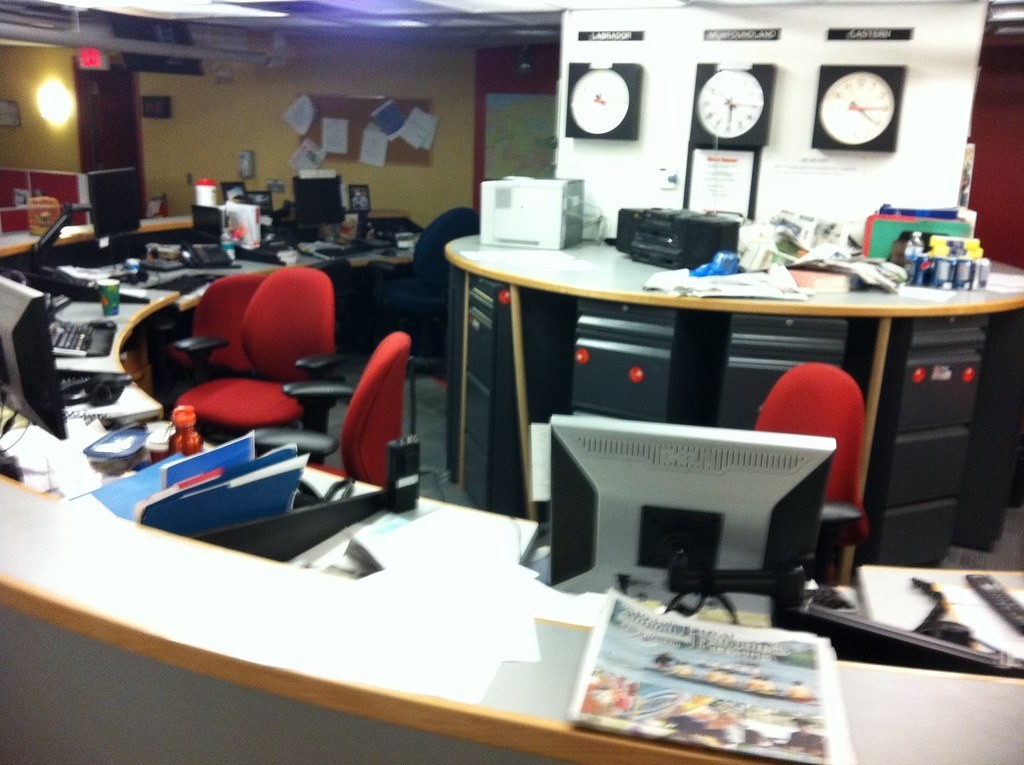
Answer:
[195,179,217,207]
[144,430,172,464]
[97,278,120,317]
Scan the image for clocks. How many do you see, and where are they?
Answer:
[811,67,909,158]
[563,58,640,142]
[686,64,777,154]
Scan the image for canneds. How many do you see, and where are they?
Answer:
[909,254,991,292]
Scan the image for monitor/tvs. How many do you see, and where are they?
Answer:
[0,276,67,441]
[293,174,345,226]
[547,415,837,615]
[86,166,142,237]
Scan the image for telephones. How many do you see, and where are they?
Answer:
[238,149,254,178]
[179,239,232,267]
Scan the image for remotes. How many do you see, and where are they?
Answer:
[967,573,1023,635]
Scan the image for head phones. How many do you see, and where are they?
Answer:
[62,375,125,408]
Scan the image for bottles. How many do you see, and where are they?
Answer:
[220,228,236,260]
[168,409,204,457]
[901,231,925,284]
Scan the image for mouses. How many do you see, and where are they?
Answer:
[89,319,114,330]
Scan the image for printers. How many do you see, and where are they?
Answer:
[617,207,739,271]
[480,176,585,249]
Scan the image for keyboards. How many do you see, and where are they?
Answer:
[51,320,115,355]
[141,259,186,272]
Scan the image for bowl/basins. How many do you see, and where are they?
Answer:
[82,427,148,477]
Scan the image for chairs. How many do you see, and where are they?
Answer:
[163,271,267,372]
[249,331,410,481]
[373,202,482,357]
[179,267,329,433]
[747,363,870,579]
